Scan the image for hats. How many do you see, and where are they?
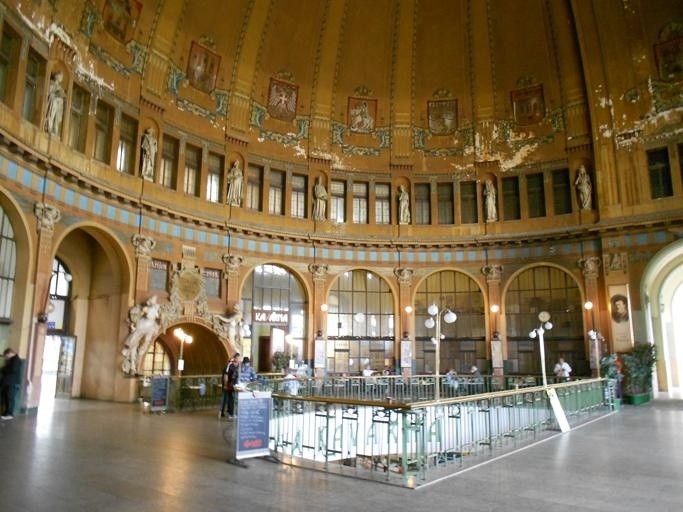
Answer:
[242,357,251,363]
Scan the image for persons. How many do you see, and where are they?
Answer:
[551,355,572,383]
[442,367,459,399]
[216,353,241,421]
[481,178,496,221]
[225,158,244,207]
[141,124,158,179]
[0,347,22,421]
[573,163,592,209]
[271,368,298,412]
[312,175,327,221]
[467,364,484,397]
[43,69,67,137]
[361,366,378,392]
[396,183,410,223]
[236,357,257,383]
[612,298,628,324]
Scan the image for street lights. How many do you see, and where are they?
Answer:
[585,300,602,377]
[172,325,194,376]
[490,304,502,341]
[527,311,553,385]
[285,333,296,359]
[424,303,458,402]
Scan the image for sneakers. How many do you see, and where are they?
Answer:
[230,415,237,418]
[218,410,222,418]
